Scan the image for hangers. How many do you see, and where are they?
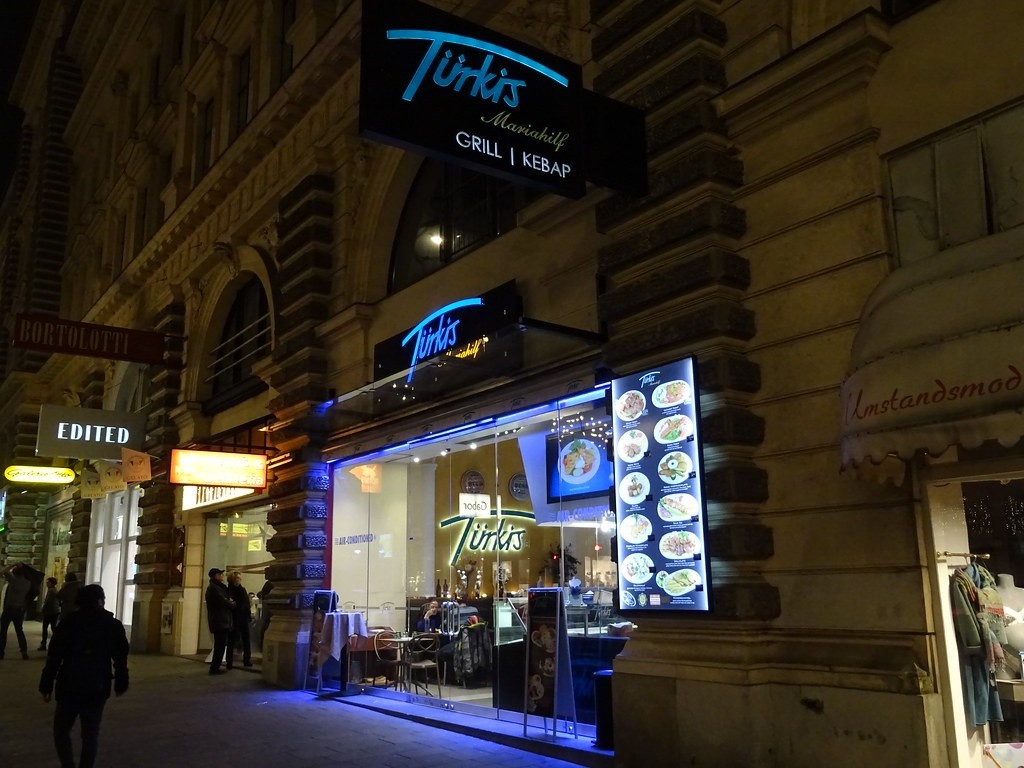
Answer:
[949,553,997,584]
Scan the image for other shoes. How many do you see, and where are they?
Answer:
[245,659,253,666]
[226,663,233,670]
[22,652,29,659]
[38,644,46,650]
[0,652,5,660]
[208,670,227,675]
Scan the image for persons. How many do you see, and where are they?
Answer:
[0,561,45,659]
[256,567,273,651]
[205,568,254,675]
[38,573,85,651]
[248,592,260,624]
[417,592,478,683]
[39,585,129,768]
[996,574,1024,679]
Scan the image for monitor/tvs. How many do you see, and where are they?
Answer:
[547,420,615,503]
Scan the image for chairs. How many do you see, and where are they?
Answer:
[374,629,442,699]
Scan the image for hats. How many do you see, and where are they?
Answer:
[208,568,225,577]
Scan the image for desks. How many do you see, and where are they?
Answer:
[322,612,368,699]
[379,638,434,692]
